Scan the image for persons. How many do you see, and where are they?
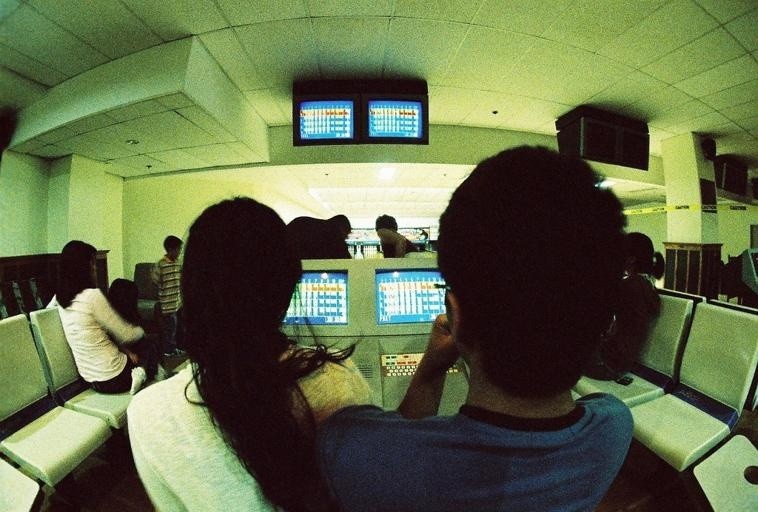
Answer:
[56,240,169,394]
[126,197,377,512]
[150,236,186,357]
[289,214,353,259]
[376,214,417,258]
[317,144,667,511]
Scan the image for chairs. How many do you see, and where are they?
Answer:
[569,262,757,512]
[0,254,168,512]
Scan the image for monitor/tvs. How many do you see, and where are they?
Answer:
[281,270,348,325]
[347,244,356,258]
[376,268,448,325]
[362,245,383,259]
[362,93,428,144]
[293,93,361,146]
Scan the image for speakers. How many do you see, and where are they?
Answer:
[556,116,619,167]
[714,162,747,197]
[619,126,649,171]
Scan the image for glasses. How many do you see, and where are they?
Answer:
[433,284,447,305]
